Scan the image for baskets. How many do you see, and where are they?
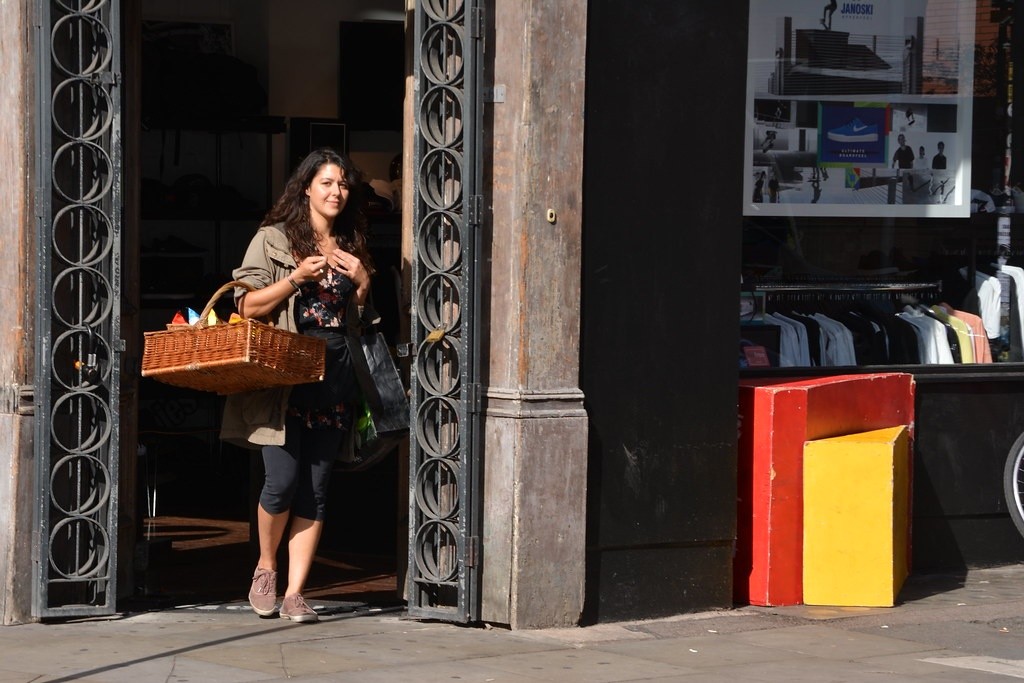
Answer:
[141,281,327,397]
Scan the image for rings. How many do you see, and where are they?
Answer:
[320,268,323,274]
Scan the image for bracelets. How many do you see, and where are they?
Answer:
[287,275,300,291]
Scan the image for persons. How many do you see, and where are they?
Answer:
[891,134,914,169]
[821,1,837,30]
[913,145,931,169]
[219,149,377,622]
[752,171,766,202]
[932,141,947,169]
[767,173,780,203]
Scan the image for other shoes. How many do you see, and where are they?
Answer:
[249,563,277,616]
[279,593,318,622]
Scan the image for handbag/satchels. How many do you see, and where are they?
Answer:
[337,331,410,476]
